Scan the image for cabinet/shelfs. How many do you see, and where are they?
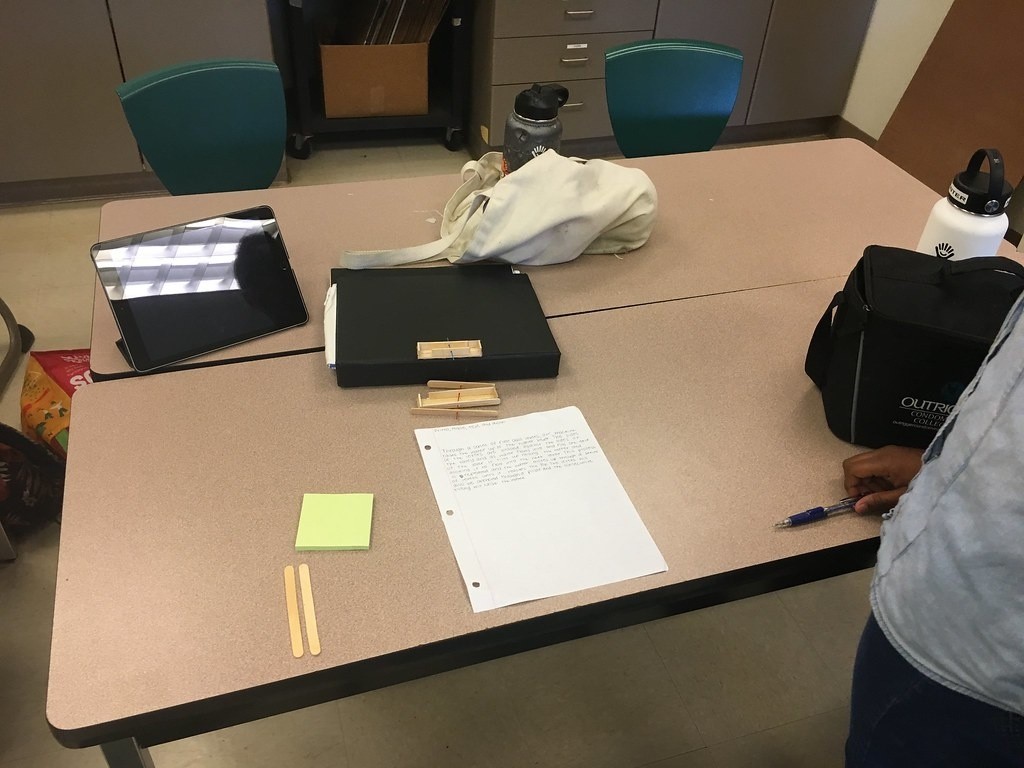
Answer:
[654,0,876,127]
[0,0,272,183]
[468,0,659,159]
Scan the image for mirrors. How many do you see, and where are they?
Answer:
[90,205,310,371]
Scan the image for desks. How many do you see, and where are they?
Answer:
[45,252,1024,768]
[92,138,1016,380]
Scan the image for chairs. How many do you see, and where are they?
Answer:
[604,37,743,158]
[116,57,287,196]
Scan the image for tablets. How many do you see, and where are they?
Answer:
[90,204,310,373]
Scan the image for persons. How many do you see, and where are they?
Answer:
[842,292,1024,768]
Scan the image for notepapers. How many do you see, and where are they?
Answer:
[294,493,375,551]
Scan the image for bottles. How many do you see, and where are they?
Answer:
[502,83,569,179]
[916,147,1014,261]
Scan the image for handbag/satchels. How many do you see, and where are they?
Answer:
[804,244,1024,449]
[20,348,93,454]
[340,151,658,265]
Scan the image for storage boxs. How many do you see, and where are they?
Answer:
[329,262,560,387]
[317,40,429,119]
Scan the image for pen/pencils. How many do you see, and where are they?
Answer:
[771,489,877,528]
